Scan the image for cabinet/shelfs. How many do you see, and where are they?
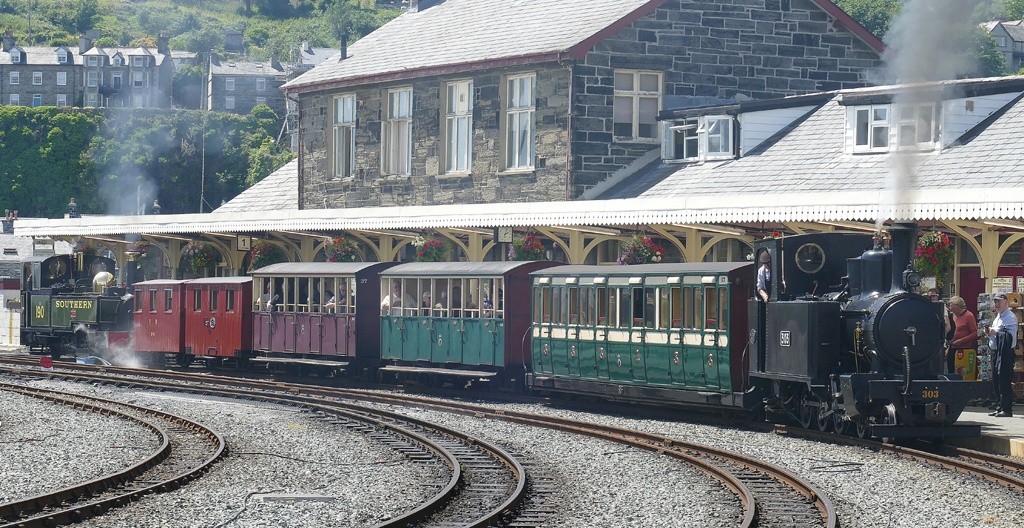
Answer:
[976,292,1024,402]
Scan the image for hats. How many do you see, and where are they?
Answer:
[760,251,771,264]
[423,291,432,298]
[993,293,1007,300]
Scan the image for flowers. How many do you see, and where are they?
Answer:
[134,241,153,265]
[412,234,447,261]
[322,236,356,262]
[764,231,783,240]
[185,240,218,280]
[247,240,278,269]
[617,232,665,265]
[83,247,97,256]
[508,232,547,261]
[911,230,955,287]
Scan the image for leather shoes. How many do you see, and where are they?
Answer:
[995,411,1013,417]
[990,410,998,416]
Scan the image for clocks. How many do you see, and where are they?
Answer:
[494,226,513,243]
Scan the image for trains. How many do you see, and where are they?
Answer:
[21,220,991,444]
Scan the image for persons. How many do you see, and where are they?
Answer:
[756,250,773,303]
[985,293,1018,417]
[382,281,502,318]
[929,289,976,374]
[257,280,355,313]
